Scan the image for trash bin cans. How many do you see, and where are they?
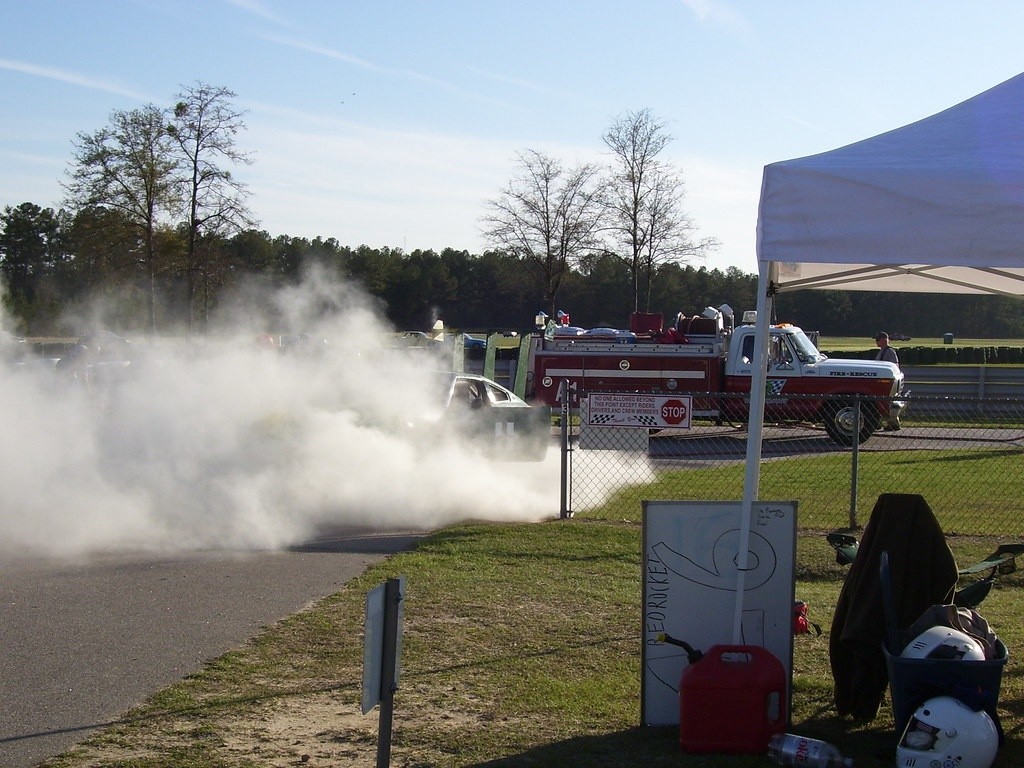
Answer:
[879,628,1009,747]
[944,333,953,343]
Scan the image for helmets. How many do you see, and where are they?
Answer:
[896,695,999,768]
[902,626,987,661]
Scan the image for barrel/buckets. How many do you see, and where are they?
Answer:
[655,631,785,755]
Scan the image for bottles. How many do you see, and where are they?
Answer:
[768,732,853,768]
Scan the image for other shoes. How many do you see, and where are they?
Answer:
[884,424,901,431]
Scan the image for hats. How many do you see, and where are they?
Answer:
[872,332,888,339]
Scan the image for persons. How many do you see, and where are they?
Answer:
[872,332,901,431]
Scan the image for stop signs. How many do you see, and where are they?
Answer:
[661,399,688,427]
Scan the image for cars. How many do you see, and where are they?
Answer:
[446,331,488,351]
[397,330,443,348]
[412,371,552,462]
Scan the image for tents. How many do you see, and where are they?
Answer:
[733,72,1024,659]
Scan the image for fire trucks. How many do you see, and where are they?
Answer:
[528,305,911,448]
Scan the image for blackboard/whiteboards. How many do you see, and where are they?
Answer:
[640,500,800,728]
